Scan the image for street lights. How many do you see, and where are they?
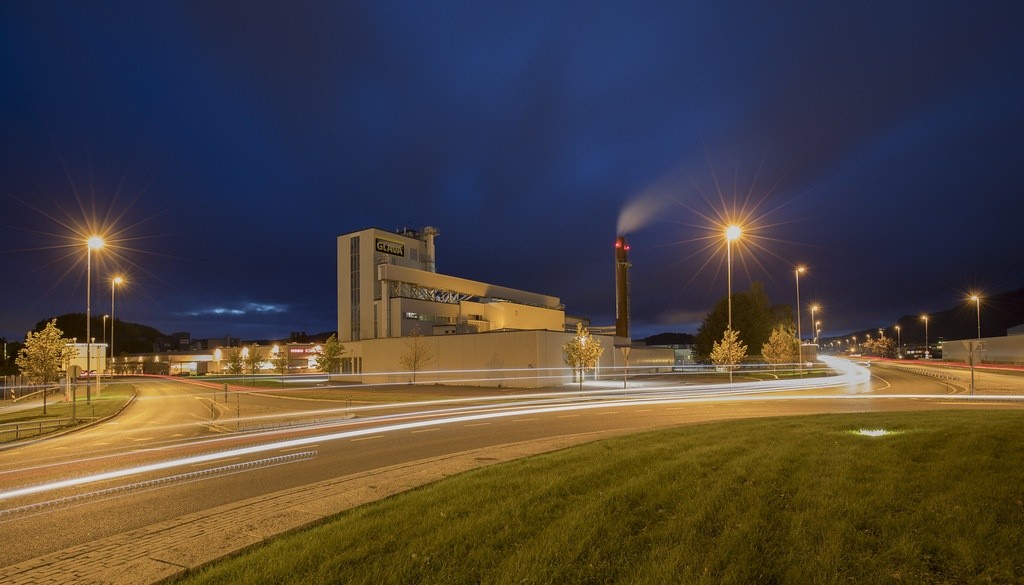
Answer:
[213,344,280,387]
[812,306,821,344]
[83,234,106,406]
[722,219,745,383]
[103,314,109,344]
[972,294,986,364]
[823,336,857,352]
[111,274,124,381]
[879,331,884,358]
[920,312,930,359]
[894,325,901,355]
[866,334,871,356]
[574,336,585,391]
